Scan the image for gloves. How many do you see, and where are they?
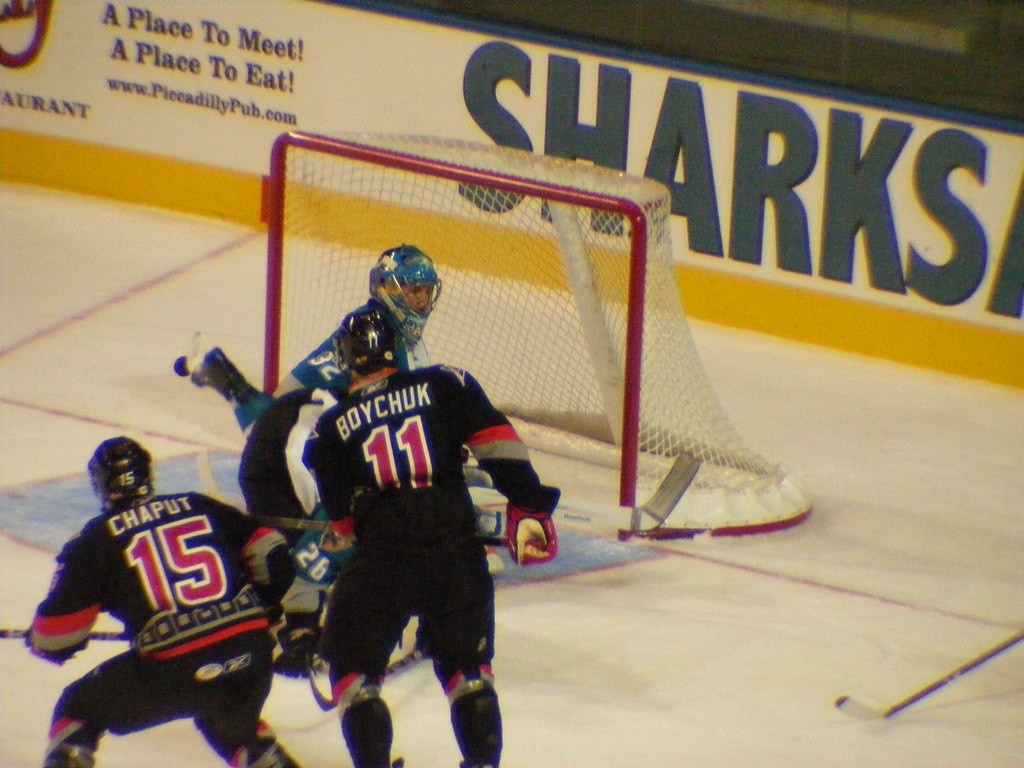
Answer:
[272,621,323,677]
[504,503,557,565]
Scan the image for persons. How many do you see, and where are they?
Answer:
[24,436,296,768]
[190,244,561,768]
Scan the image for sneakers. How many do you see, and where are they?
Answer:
[183,328,246,397]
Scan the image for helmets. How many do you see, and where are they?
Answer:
[369,243,443,344]
[334,307,398,375]
[87,436,155,507]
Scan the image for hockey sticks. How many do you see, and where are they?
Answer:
[835,629,1023,725]
[465,449,704,535]
[306,647,429,714]
[0,627,127,642]
[194,444,547,553]
